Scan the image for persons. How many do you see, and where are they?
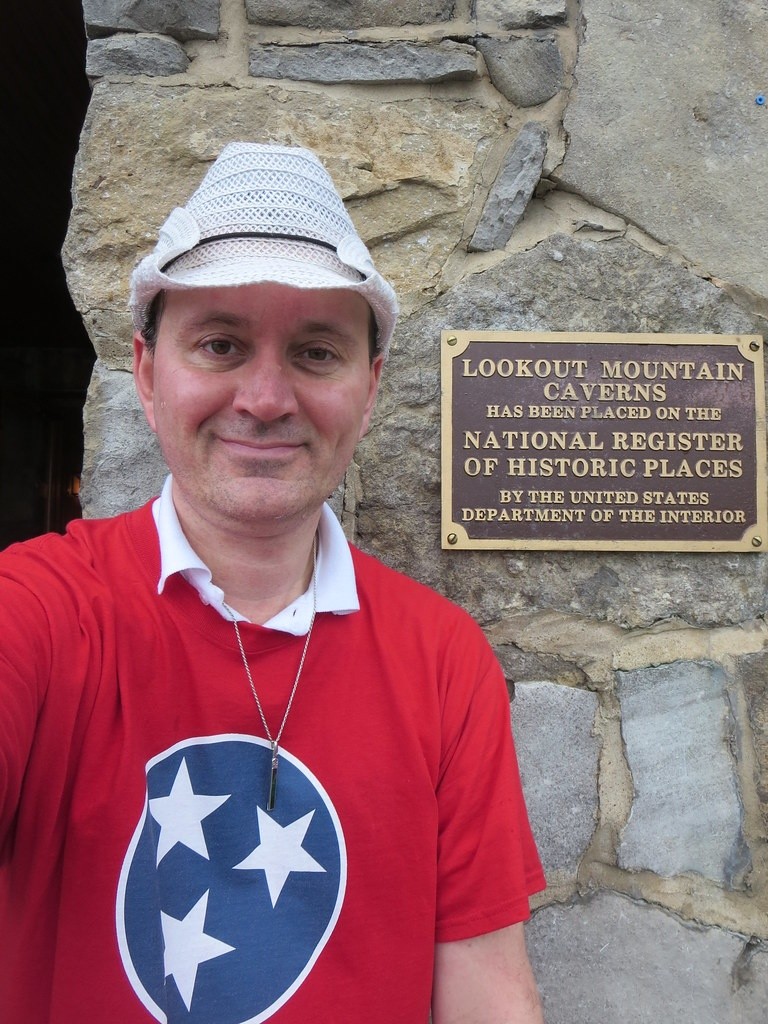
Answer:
[0,144,548,1024]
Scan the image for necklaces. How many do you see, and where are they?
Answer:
[225,541,318,810]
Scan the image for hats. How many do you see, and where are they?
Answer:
[126,140,399,363]
